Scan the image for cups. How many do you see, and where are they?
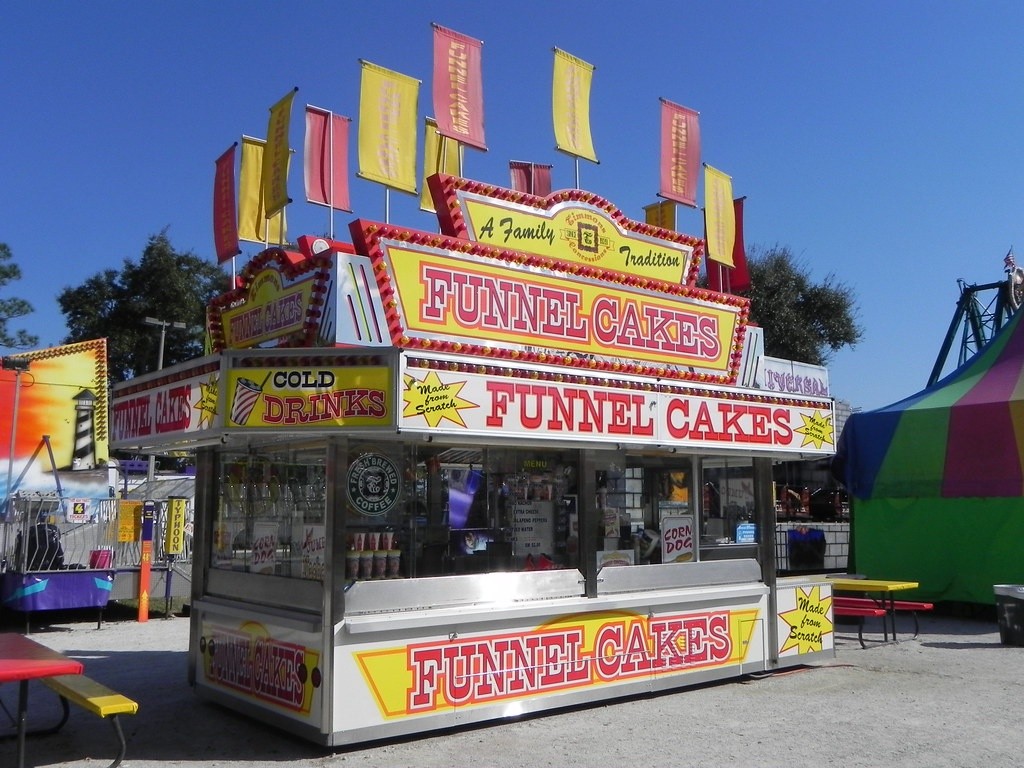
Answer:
[228,377,262,427]
[346,526,401,580]
[517,483,553,500]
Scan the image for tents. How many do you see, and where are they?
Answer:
[832,302,1024,606]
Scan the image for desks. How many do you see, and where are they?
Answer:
[828,577,921,650]
[0,634,126,768]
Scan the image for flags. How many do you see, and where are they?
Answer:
[1004,249,1013,263]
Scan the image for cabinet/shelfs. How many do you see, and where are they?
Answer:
[400,446,503,577]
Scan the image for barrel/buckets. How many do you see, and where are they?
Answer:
[91,550,112,568]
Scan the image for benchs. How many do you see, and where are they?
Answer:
[833,607,886,617]
[832,597,933,610]
[40,674,137,718]
